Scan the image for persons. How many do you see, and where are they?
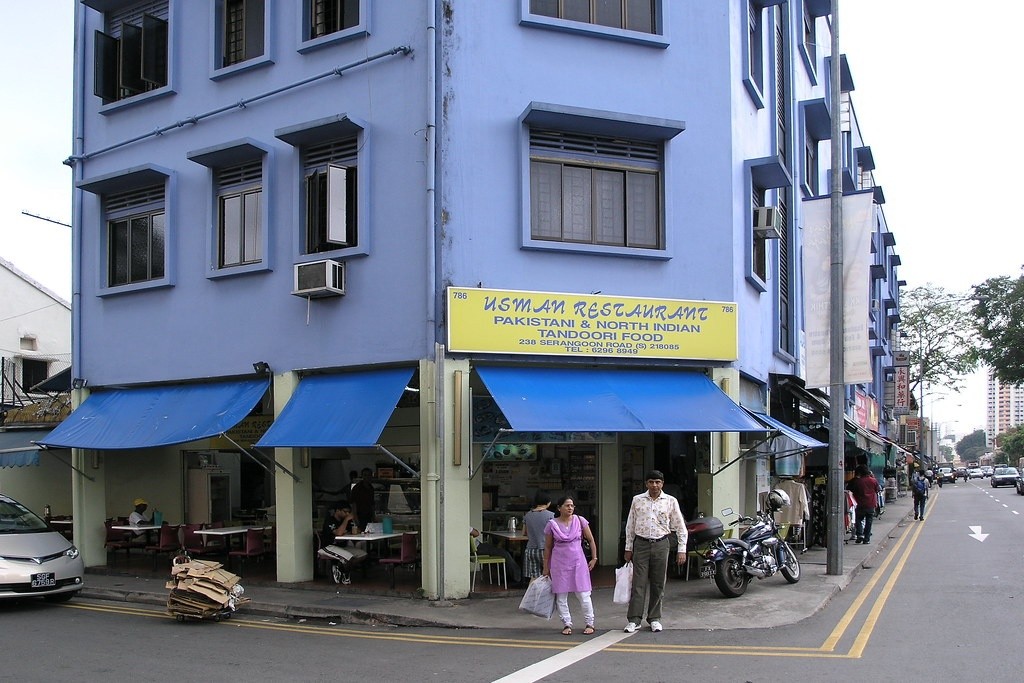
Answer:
[319,501,369,585]
[937,469,943,488]
[925,467,934,488]
[623,469,689,633]
[911,470,929,521]
[846,462,880,544]
[541,495,599,635]
[350,467,375,530]
[128,497,155,536]
[470,528,529,588]
[963,469,968,482]
[522,488,556,579]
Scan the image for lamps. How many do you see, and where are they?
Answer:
[721,378,730,463]
[453,369,462,466]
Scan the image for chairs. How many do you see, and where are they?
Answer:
[145,524,180,570]
[228,528,265,578]
[773,521,792,541]
[316,528,336,583]
[686,529,733,582]
[105,520,131,570]
[183,523,207,562]
[470,535,507,592]
[379,533,418,590]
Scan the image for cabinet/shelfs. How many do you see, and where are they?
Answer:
[570,453,597,491]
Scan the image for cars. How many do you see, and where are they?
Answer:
[990,468,1022,489]
[0,494,85,606]
[938,467,957,483]
[980,464,1011,478]
[968,469,983,479]
[1015,468,1024,494]
[956,467,968,477]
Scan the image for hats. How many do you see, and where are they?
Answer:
[134,498,148,506]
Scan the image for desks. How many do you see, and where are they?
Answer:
[481,529,530,559]
[334,529,419,560]
[112,525,181,545]
[193,524,273,564]
[51,519,72,533]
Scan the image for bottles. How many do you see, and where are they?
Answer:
[384,512,392,533]
[393,457,420,479]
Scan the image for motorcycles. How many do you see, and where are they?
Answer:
[686,487,802,600]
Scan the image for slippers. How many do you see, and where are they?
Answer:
[583,624,595,635]
[562,626,571,635]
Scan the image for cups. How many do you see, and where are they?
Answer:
[508,516,515,533]
[352,526,358,534]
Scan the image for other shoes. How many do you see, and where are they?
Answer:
[920,518,924,521]
[855,536,863,543]
[914,513,918,520]
[863,541,870,544]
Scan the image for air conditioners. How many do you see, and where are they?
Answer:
[906,431,917,446]
[290,258,346,301]
[872,298,880,312]
[752,205,784,241]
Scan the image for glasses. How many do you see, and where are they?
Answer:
[560,504,575,508]
[342,509,351,514]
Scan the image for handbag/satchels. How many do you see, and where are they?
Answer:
[613,560,634,604]
[519,573,557,620]
[577,515,592,563]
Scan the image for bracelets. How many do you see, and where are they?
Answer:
[593,558,597,560]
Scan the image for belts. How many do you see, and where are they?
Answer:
[635,535,667,543]
[137,531,145,537]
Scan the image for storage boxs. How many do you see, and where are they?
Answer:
[685,516,725,546]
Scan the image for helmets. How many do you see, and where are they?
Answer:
[765,488,790,512]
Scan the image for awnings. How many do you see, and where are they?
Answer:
[0,430,57,468]
[740,404,829,460]
[29,374,275,482]
[249,367,419,483]
[467,363,781,479]
[780,378,914,464]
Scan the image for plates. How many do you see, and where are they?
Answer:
[493,450,504,460]
[502,450,509,456]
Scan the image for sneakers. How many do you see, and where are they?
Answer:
[341,573,350,584]
[650,621,662,632]
[624,621,641,632]
[332,565,341,584]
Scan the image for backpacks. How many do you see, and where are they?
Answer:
[914,477,925,495]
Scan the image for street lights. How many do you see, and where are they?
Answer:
[917,296,982,471]
[939,420,960,465]
[930,404,962,470]
